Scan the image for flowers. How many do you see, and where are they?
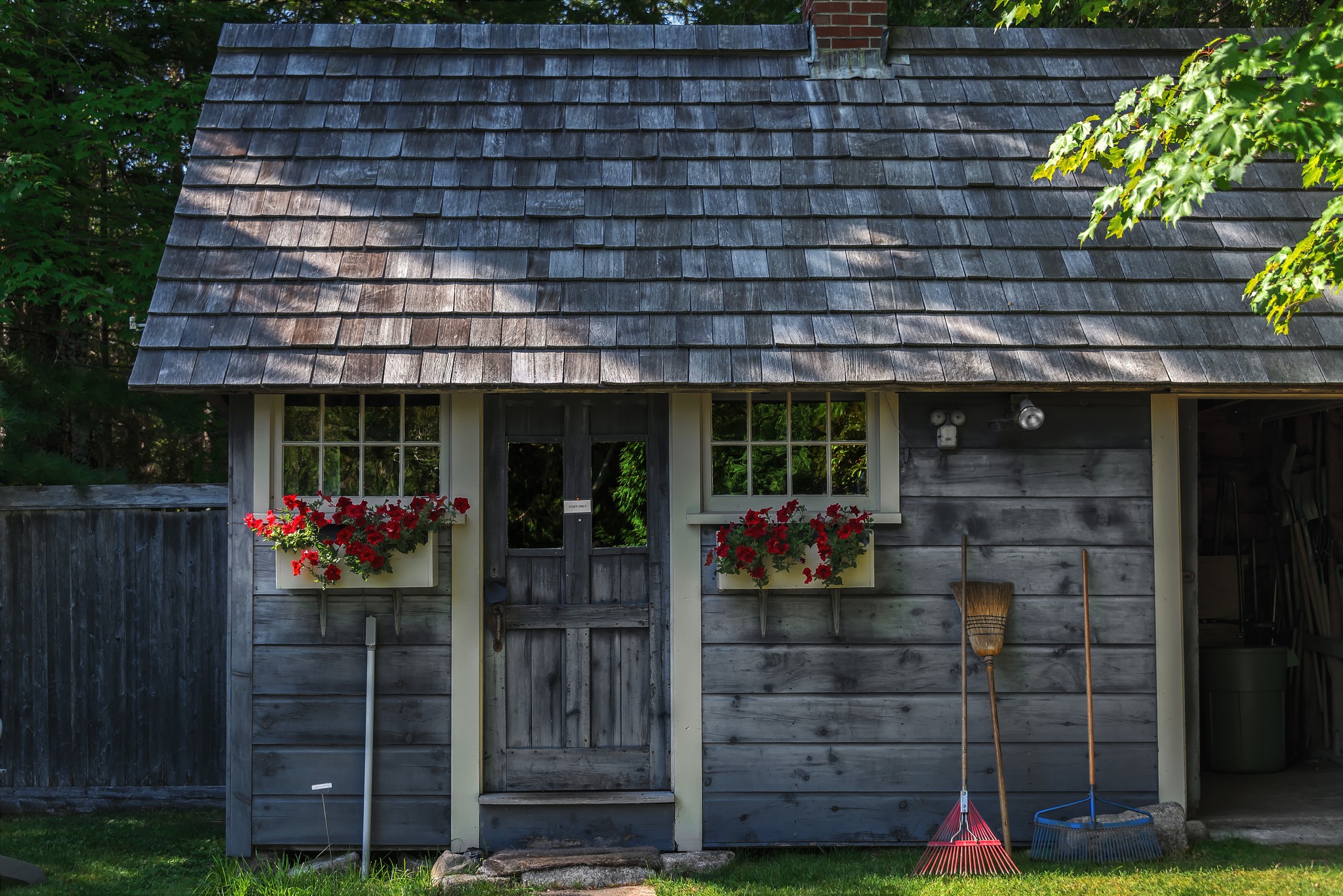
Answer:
[243,490,470,590]
[704,497,874,597]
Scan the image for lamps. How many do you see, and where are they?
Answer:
[1013,399,1045,430]
[929,409,968,450]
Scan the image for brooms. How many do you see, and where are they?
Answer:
[951,580,1013,859]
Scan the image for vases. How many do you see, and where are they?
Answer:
[716,528,874,589]
[276,530,438,589]
